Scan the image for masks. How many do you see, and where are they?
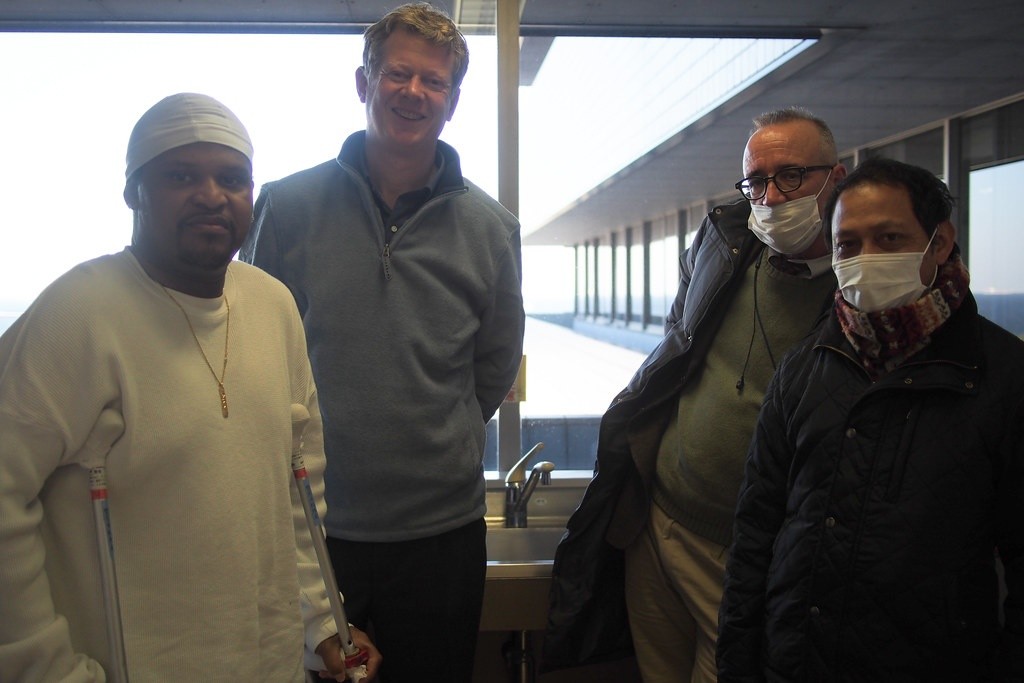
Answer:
[748,169,834,255]
[832,224,942,313]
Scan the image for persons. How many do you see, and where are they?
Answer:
[235,0,528,682]
[3,89,386,683]
[714,151,1024,683]
[548,104,852,683]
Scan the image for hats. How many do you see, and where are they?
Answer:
[125,92,254,184]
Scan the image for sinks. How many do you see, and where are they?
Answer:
[478,529,565,631]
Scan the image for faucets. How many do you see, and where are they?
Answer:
[503,441,554,528]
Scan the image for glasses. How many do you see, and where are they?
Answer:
[735,165,835,200]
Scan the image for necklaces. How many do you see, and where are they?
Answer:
[138,259,231,419]
